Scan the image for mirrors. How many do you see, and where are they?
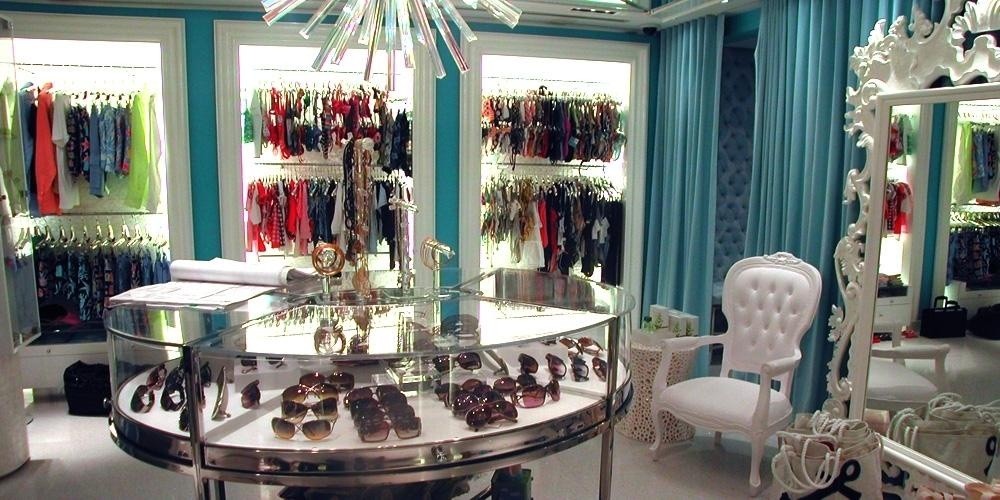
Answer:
[816,0,1000,500]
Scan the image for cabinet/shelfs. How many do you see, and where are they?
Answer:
[457,29,650,321]
[872,102,935,334]
[100,267,638,500]
[0,8,210,402]
[930,98,1000,329]
[195,3,448,339]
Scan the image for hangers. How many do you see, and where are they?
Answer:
[0,63,158,107]
[948,203,1000,236]
[479,76,627,110]
[956,103,1000,131]
[247,67,407,104]
[10,212,168,257]
[479,161,622,205]
[885,166,910,190]
[247,160,412,194]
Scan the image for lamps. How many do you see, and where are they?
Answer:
[257,0,524,92]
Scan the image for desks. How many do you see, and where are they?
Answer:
[619,328,698,444]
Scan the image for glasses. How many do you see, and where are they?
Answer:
[561,336,608,384]
[432,351,567,432]
[130,357,261,443]
[271,371,423,444]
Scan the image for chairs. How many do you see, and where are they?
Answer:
[645,251,825,499]
[860,317,951,448]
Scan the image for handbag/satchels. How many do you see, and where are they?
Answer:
[62,360,113,419]
[769,409,886,500]
[919,295,968,340]
[886,390,999,484]
